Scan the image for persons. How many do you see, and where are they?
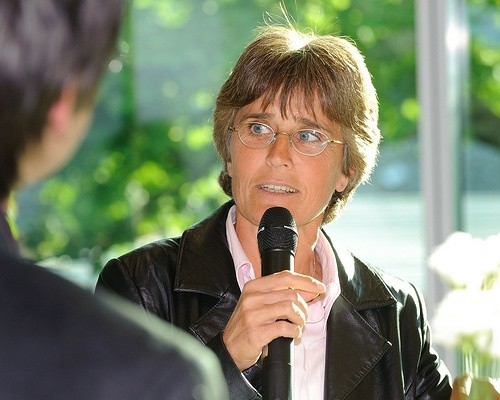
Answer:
[93,1,500,400]
[0,0,230,400]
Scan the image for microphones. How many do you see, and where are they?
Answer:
[257,206,299,400]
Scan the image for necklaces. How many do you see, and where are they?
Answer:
[311,255,317,279]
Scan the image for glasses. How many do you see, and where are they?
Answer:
[230,122,347,157]
